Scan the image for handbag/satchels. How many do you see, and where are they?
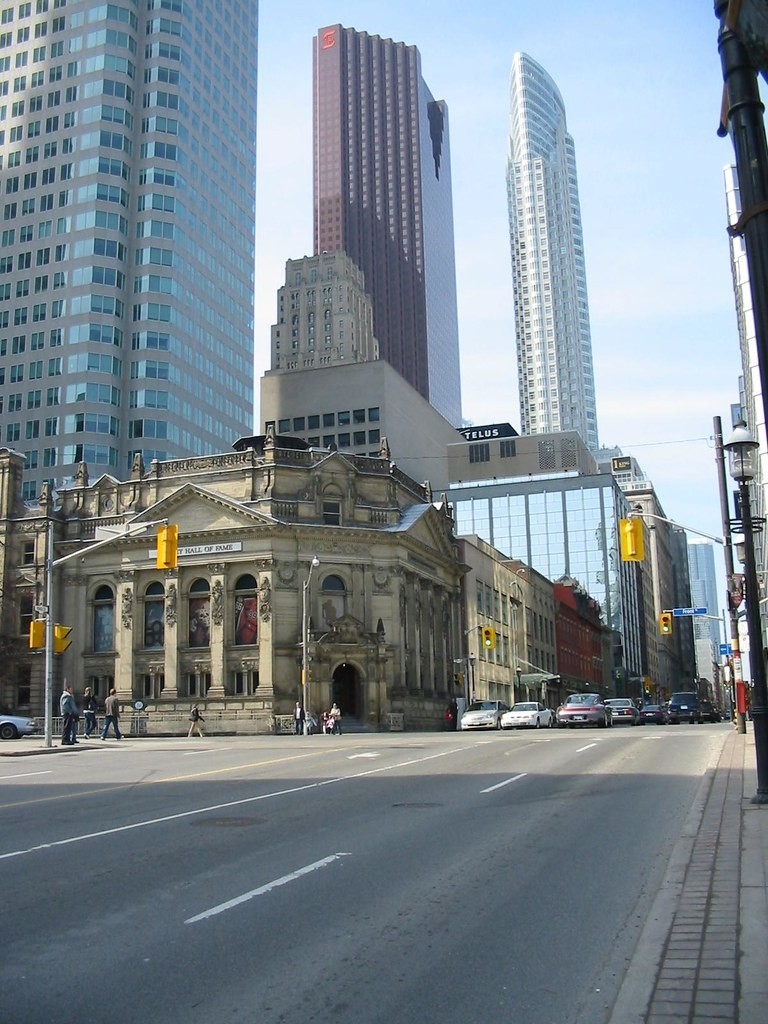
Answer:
[189,717,197,721]
[335,716,342,720]
[88,701,96,709]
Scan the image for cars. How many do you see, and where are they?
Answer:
[501,702,554,729]
[461,699,511,730]
[604,698,641,726]
[558,694,613,728]
[0,714,41,739]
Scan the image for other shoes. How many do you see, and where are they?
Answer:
[84,733,89,739]
[73,740,80,743]
[62,742,74,745]
[118,736,125,740]
[100,736,104,740]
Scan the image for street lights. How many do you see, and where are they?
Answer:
[468,652,477,701]
[724,418,768,805]
[516,666,522,702]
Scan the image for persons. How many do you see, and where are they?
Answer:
[188,703,204,737]
[100,689,124,741]
[82,686,98,740]
[293,701,306,735]
[445,697,457,729]
[320,702,342,735]
[306,712,317,736]
[60,686,80,745]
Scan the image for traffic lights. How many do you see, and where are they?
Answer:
[302,555,320,736]
[54,625,74,655]
[454,673,462,687]
[482,627,497,649]
[660,613,672,635]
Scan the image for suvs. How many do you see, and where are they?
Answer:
[640,705,666,725]
[665,692,704,725]
[700,699,730,723]
